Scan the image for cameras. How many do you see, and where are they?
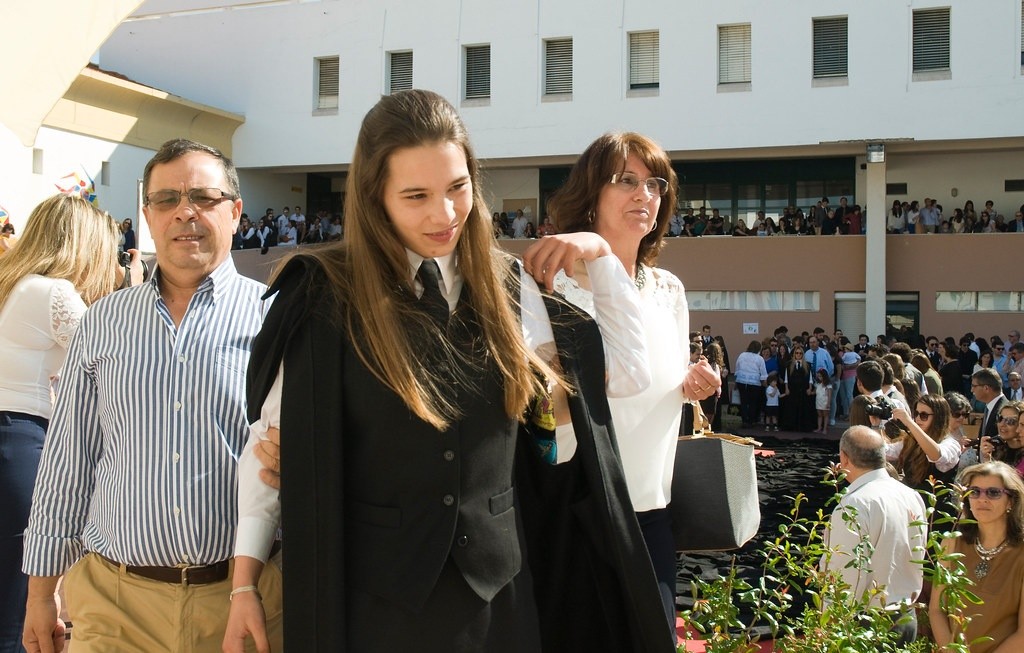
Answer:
[865,400,892,420]
[117,251,148,285]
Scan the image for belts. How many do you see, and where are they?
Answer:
[95,541,281,583]
[1012,390,1018,401]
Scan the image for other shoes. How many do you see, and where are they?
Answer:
[764,426,769,432]
[773,426,779,431]
[830,420,835,425]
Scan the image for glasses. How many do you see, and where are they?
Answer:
[796,352,803,355]
[931,343,938,347]
[607,172,668,197]
[125,222,130,225]
[996,414,1018,426]
[952,412,970,419]
[914,410,934,421]
[966,486,1012,499]
[996,348,1004,351]
[771,344,778,347]
[146,188,236,212]
[962,344,970,347]
[694,340,704,343]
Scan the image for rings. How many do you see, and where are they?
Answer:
[704,385,712,391]
[692,389,701,393]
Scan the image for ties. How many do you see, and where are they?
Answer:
[813,353,816,377]
[797,361,800,369]
[418,262,450,337]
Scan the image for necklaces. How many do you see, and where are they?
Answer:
[973,535,1009,581]
[634,264,645,289]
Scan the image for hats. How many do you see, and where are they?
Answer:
[845,343,855,351]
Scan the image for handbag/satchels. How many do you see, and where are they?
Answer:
[668,401,762,552]
[514,256,579,468]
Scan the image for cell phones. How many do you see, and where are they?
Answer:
[987,436,1007,450]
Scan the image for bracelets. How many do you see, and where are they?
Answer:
[229,585,262,601]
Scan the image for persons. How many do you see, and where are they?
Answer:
[22,138,286,653]
[220,89,671,653]
[686,325,1024,653]
[487,209,557,239]
[116,218,135,258]
[231,206,345,254]
[539,130,723,645]
[0,223,15,253]
[0,192,149,653]
[666,197,1024,236]
